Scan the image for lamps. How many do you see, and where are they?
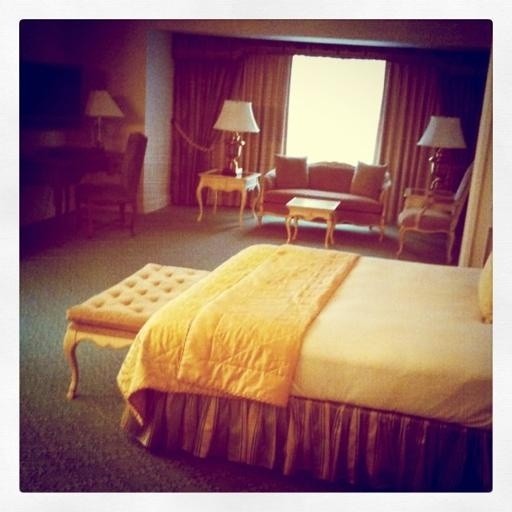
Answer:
[81,89,125,145]
[213,100,260,176]
[416,115,467,195]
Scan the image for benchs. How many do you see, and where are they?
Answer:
[62,262,213,400]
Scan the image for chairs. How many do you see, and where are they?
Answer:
[396,160,474,263]
[77,132,148,238]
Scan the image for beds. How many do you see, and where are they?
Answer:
[116,242,493,493]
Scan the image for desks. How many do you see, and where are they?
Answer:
[20,148,122,247]
[402,187,455,209]
[196,169,261,227]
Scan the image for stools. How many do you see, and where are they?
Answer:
[285,197,341,249]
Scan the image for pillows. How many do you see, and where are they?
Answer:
[478,251,493,323]
[273,153,387,200]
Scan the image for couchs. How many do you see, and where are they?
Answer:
[258,169,392,243]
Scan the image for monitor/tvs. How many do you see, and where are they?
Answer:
[20,63,81,129]
